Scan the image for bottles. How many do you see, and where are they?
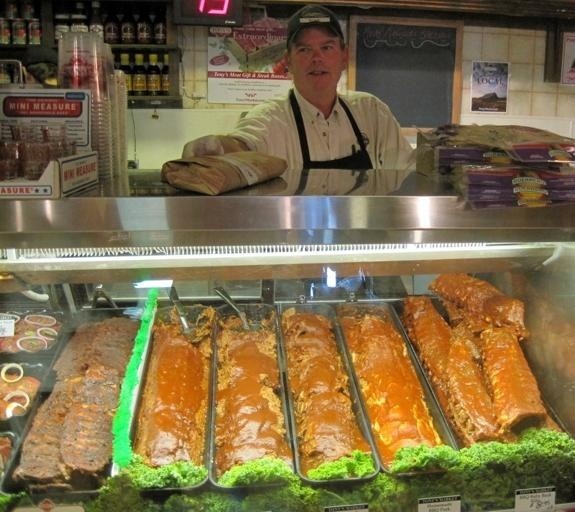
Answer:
[72,0,169,96]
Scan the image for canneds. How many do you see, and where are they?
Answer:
[0,1,166,46]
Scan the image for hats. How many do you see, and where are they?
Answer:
[287,4,344,48]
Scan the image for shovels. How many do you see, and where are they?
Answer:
[166,287,208,342]
[214,286,260,333]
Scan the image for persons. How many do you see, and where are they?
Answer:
[182,4,414,299]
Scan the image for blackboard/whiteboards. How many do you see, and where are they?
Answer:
[346,15,464,136]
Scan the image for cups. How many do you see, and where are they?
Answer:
[58,31,128,184]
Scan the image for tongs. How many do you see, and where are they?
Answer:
[92,288,117,309]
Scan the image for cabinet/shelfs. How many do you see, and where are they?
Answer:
[1,0,182,107]
[0,195,573,511]
[0,88,100,199]
